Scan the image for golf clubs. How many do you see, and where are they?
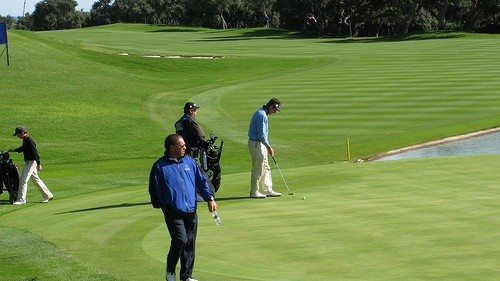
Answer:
[273,157,295,195]
[205,132,224,156]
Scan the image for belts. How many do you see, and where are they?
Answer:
[249,138,260,141]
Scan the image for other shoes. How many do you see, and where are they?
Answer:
[250,193,266,198]
[13,200,26,204]
[197,194,204,201]
[42,197,53,203]
[263,191,282,197]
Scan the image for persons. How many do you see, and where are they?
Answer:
[8,127,53,204]
[174,102,211,163]
[247,97,281,198]
[149,134,217,281]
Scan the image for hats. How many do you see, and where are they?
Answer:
[269,97,281,112]
[13,126,26,136]
[182,102,200,109]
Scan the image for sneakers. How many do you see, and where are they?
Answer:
[180,276,198,281]
[165,272,176,281]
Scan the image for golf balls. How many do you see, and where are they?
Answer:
[302,196,306,199]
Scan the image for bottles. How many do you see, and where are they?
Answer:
[211,209,222,226]
